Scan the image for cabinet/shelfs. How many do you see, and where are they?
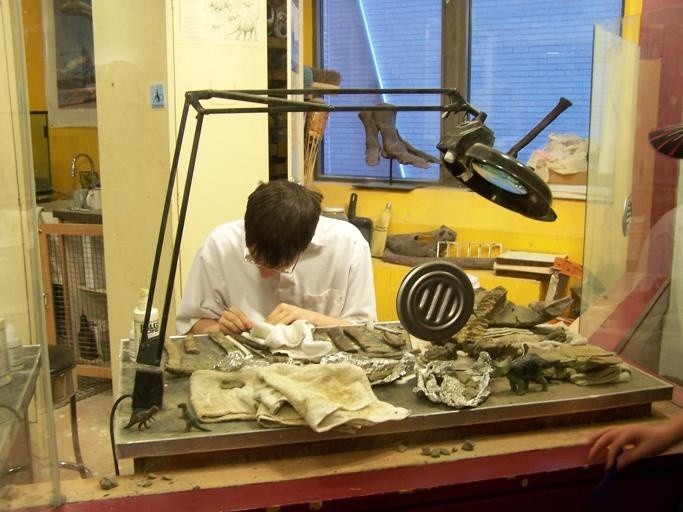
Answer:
[35,218,111,380]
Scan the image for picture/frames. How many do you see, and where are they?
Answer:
[40,0,96,129]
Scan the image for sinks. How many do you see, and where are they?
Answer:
[53,208,102,224]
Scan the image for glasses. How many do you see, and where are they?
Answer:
[242,245,301,275]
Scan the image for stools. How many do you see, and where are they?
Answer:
[40,343,87,480]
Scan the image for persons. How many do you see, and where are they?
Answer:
[175,177,378,337]
[585,405,682,470]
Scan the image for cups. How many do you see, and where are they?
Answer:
[78,171,98,190]
[85,187,101,210]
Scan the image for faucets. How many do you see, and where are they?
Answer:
[73,154,95,196]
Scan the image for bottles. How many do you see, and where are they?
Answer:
[128,288,160,362]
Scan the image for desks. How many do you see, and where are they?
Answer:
[114,322,673,476]
